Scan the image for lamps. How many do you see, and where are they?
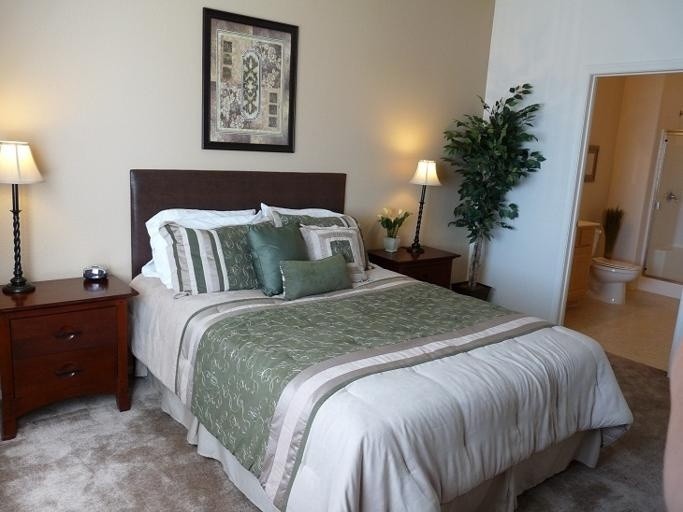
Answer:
[0,141,47,294]
[407,159,442,253]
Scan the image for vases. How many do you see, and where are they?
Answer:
[384,236,401,252]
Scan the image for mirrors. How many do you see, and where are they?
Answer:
[584,145,599,183]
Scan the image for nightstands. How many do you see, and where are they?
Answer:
[0,274,140,441]
[367,246,462,289]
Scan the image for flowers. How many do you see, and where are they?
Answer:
[377,208,414,237]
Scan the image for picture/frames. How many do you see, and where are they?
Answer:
[202,7,299,153]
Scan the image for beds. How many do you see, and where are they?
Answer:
[130,168,634,512]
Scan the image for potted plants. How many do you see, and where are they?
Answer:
[440,84,547,302]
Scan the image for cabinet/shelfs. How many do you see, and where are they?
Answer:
[567,219,599,307]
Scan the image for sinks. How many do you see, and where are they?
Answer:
[578,221,600,227]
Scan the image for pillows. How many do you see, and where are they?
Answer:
[280,254,354,300]
[300,223,368,282]
[260,203,343,222]
[246,220,310,296]
[145,208,257,287]
[273,211,369,272]
[159,221,276,299]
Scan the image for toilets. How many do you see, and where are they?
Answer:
[586,229,641,305]
